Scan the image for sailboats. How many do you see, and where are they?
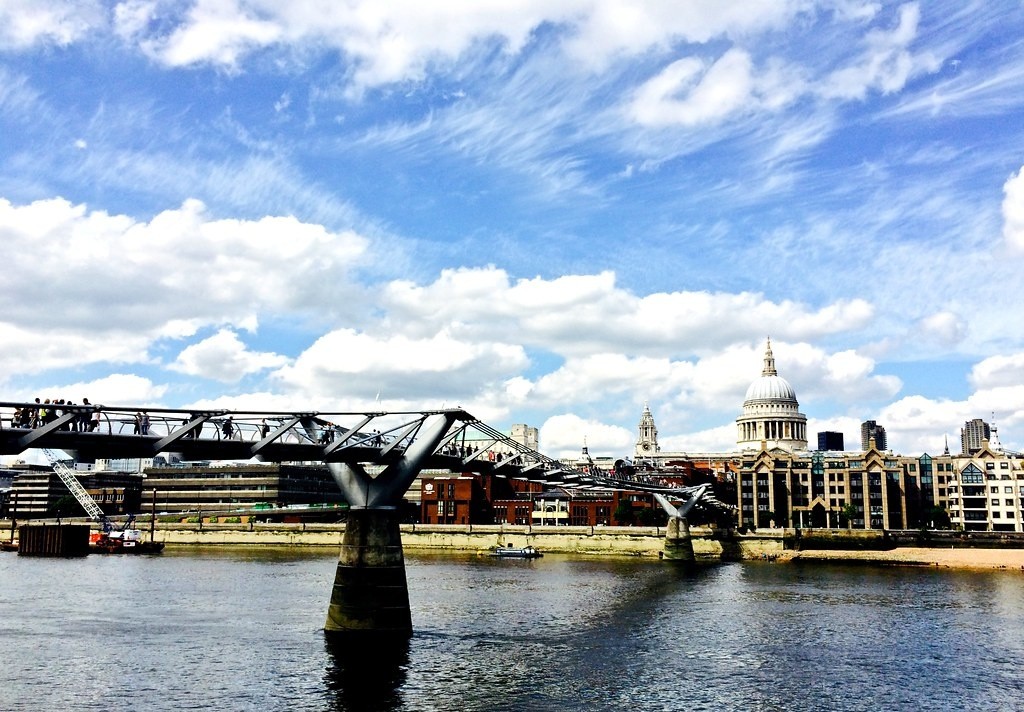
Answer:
[90,511,165,554]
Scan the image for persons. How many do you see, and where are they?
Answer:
[141,411,151,435]
[222,416,234,440]
[438,441,679,489]
[261,418,269,438]
[369,429,382,447]
[317,422,339,445]
[134,413,142,434]
[12,397,103,432]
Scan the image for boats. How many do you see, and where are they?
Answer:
[489,547,543,559]
